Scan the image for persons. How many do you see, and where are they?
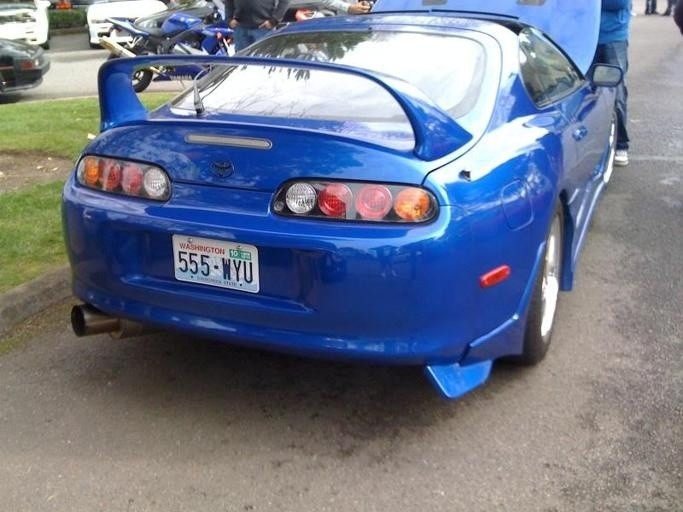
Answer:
[583,0,630,167]
[643,0,660,14]
[323,0,375,15]
[225,0,291,55]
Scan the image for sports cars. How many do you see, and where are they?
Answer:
[62,0,623,400]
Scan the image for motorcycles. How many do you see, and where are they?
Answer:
[99,0,235,92]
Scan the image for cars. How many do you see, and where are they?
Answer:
[87,0,168,49]
[0,0,51,94]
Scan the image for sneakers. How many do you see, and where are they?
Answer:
[614,148,628,167]
